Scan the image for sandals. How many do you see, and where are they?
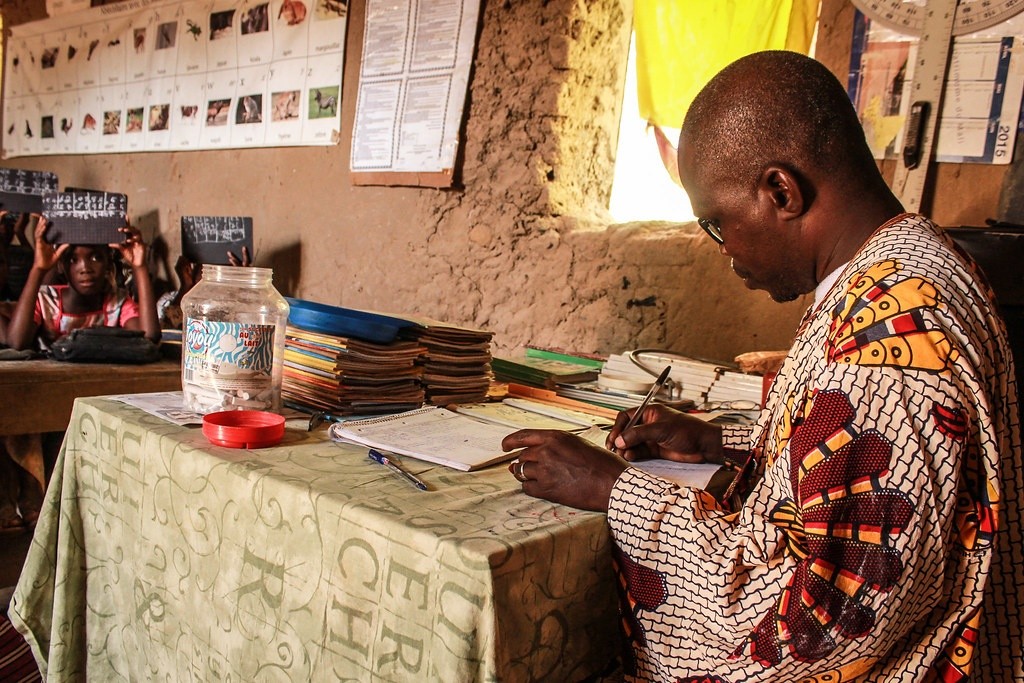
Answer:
[18,504,40,530]
[0,496,26,533]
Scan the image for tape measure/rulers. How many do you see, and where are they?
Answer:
[848,0,1024,214]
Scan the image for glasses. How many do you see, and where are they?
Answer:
[698,185,759,245]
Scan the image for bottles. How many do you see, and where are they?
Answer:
[180,264,291,417]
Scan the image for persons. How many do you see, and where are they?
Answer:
[0,211,163,530]
[502,51,1024,683]
[157,245,249,328]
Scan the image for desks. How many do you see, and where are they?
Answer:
[0,353,181,535]
[6,391,740,683]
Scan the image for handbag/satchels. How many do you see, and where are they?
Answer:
[50,326,156,365]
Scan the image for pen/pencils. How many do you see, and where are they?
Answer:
[610,365,673,452]
[368,448,429,493]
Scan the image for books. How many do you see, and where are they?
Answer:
[281,297,763,491]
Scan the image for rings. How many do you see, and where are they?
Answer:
[518,461,530,481]
